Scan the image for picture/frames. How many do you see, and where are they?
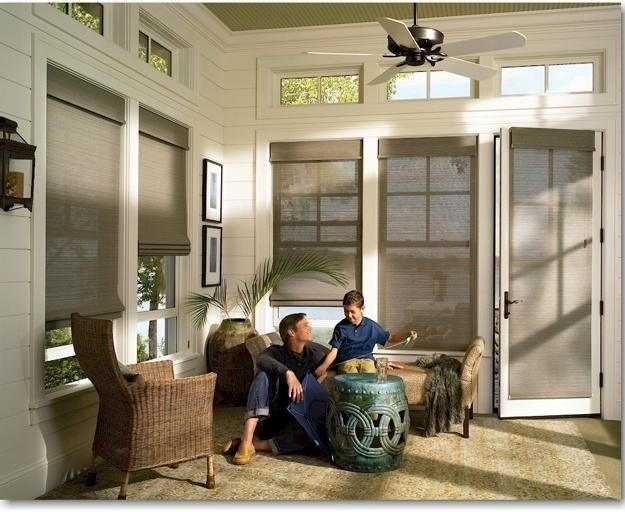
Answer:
[202,224,222,288]
[202,159,223,223]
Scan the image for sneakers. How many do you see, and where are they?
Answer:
[231,444,256,465]
[222,434,258,453]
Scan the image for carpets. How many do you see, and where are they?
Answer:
[33,407,617,500]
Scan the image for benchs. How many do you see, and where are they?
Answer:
[245,330,485,438]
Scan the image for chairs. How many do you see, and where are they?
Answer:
[70,313,218,500]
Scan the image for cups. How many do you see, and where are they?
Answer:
[375,357,388,383]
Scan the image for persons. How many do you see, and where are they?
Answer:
[313,291,418,434]
[223,312,404,465]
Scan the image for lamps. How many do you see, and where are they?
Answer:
[0,114,36,212]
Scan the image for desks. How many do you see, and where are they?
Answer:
[328,373,411,472]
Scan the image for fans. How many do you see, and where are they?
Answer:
[300,3,527,86]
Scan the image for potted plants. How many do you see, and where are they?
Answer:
[185,250,351,407]
